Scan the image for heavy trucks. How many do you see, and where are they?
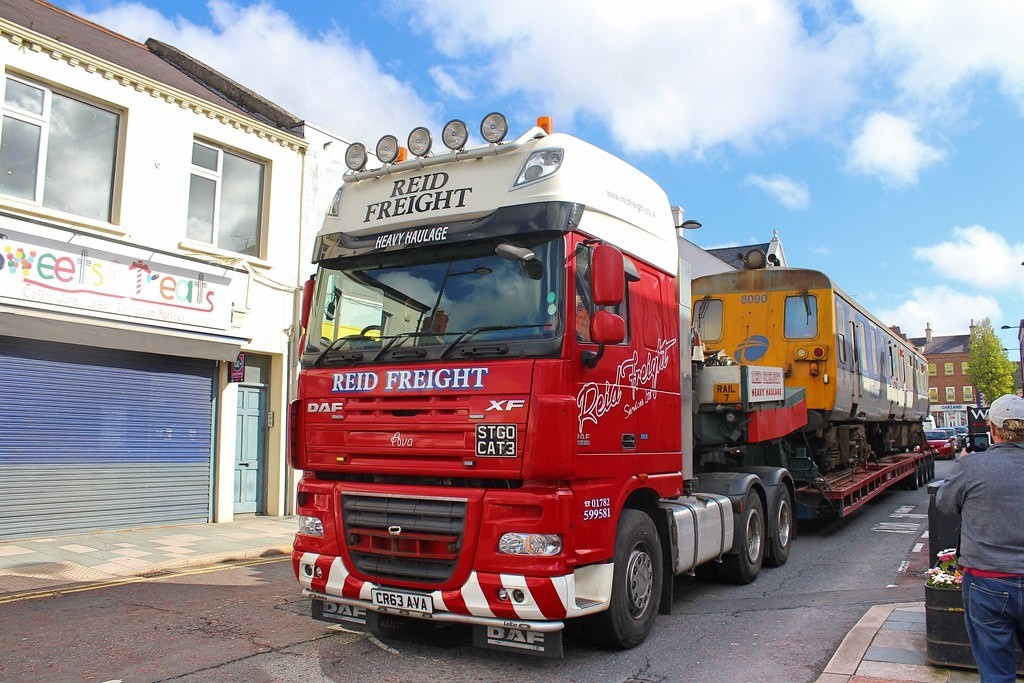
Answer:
[282,114,936,658]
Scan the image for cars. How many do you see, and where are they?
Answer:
[932,427,963,453]
[951,427,967,449]
[923,430,956,461]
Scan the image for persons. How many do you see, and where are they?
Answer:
[935,393,1024,683]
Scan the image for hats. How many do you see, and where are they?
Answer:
[989,394,1024,428]
